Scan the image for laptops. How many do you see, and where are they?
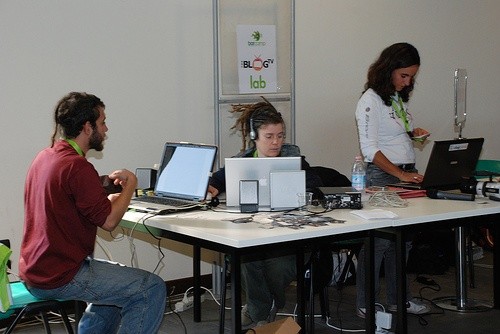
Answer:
[385,137,485,190]
[127,142,218,214]
[224,157,301,212]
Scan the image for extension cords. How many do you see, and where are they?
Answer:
[175,293,205,312]
[375,329,393,334]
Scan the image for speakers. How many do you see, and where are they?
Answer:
[135,167,157,191]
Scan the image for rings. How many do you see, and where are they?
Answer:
[412,176,417,179]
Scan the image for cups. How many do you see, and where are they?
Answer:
[296,192,313,215]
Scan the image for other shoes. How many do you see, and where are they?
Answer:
[389,301,429,314]
[257,300,278,327]
[241,303,254,325]
[354,307,366,318]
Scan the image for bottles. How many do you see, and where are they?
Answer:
[351,156,367,201]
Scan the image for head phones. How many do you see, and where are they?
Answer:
[250,106,271,140]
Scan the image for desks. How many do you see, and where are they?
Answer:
[111,186,500,334]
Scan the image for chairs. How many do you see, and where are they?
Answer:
[473,160,500,180]
[310,167,366,285]
[0,243,74,334]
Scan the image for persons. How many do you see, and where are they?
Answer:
[18,92,167,334]
[355,42,431,319]
[207,95,312,326]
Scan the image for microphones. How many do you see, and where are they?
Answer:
[426,190,475,201]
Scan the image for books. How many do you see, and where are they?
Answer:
[351,209,398,220]
